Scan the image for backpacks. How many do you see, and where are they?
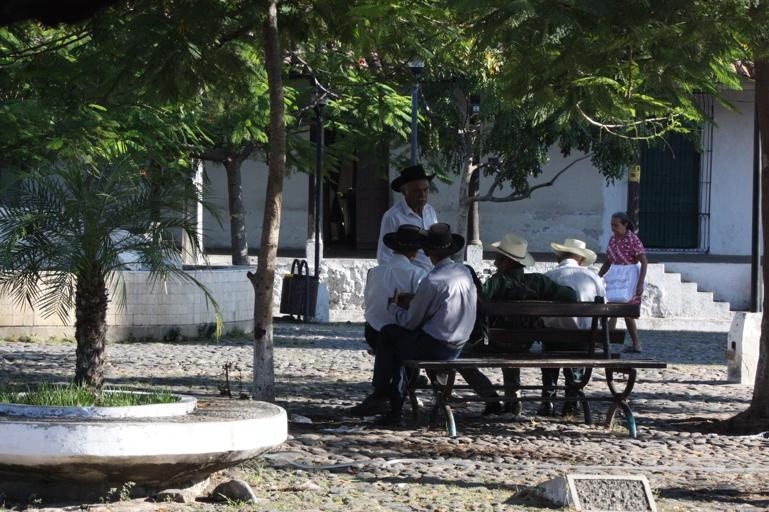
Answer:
[490,273,545,345]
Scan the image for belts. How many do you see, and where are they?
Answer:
[443,344,464,350]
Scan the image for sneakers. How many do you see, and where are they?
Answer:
[351,375,469,429]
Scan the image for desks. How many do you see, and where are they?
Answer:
[395,292,638,436]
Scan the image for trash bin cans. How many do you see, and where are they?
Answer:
[280,272,321,319]
[726,312,762,385]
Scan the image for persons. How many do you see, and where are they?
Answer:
[538,238,607,418]
[376,163,439,386]
[363,223,471,409]
[451,232,579,422]
[349,223,478,418]
[596,211,649,353]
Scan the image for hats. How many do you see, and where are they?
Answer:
[488,233,535,267]
[415,223,464,257]
[383,224,423,252]
[551,238,597,266]
[390,164,436,192]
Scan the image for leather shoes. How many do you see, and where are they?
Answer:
[483,397,582,416]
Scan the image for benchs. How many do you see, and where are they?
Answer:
[396,331,668,439]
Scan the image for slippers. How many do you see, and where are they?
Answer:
[622,346,643,354]
